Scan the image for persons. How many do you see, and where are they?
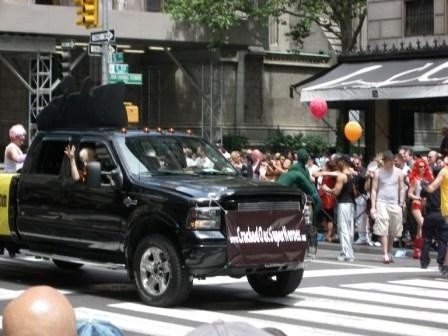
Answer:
[145,142,448,279]
[65,144,96,183]
[0,286,78,336]
[5,124,30,173]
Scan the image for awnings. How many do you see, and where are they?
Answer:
[292,53,448,104]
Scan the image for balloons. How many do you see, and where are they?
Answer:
[344,121,362,142]
[309,97,325,117]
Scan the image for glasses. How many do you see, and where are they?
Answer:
[418,166,426,168]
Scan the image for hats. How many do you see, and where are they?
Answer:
[382,150,395,160]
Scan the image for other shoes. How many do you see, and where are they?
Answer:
[336,256,353,262]
[422,264,426,268]
[412,249,421,258]
[368,241,373,246]
[354,239,367,244]
[438,261,444,268]
[384,258,389,263]
[390,257,394,263]
[415,237,424,248]
[436,270,448,281]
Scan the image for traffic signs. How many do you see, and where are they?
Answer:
[90,29,116,43]
[88,44,118,57]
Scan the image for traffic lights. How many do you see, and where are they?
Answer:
[82,0,98,27]
[58,51,72,81]
[75,0,90,29]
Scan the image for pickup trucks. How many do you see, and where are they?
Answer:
[0,124,320,310]
